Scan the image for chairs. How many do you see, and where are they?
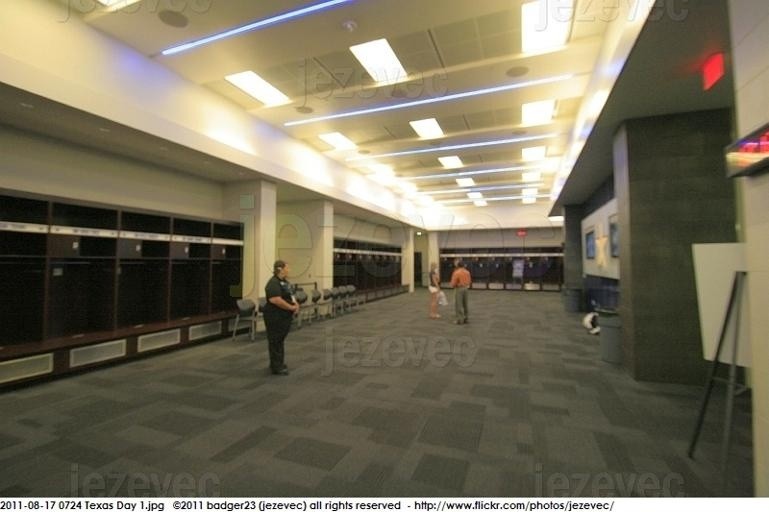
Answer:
[231,283,367,342]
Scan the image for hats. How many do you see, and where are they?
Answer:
[272,259,289,275]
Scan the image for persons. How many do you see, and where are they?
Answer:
[426,261,443,320]
[263,260,300,376]
[449,258,472,326]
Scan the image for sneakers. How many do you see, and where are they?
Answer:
[430,313,467,324]
[270,364,290,376]
